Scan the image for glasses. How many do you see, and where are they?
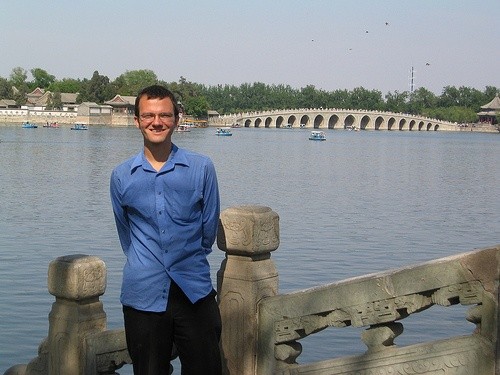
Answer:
[138,111,176,120]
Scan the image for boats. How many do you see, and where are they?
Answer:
[184,119,207,128]
[216,127,232,136]
[345,126,360,132]
[175,125,190,132]
[71,123,88,130]
[309,131,326,140]
[283,124,292,129]
[22,121,38,128]
[300,124,305,129]
[43,121,60,128]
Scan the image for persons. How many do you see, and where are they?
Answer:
[109,84,223,375]
